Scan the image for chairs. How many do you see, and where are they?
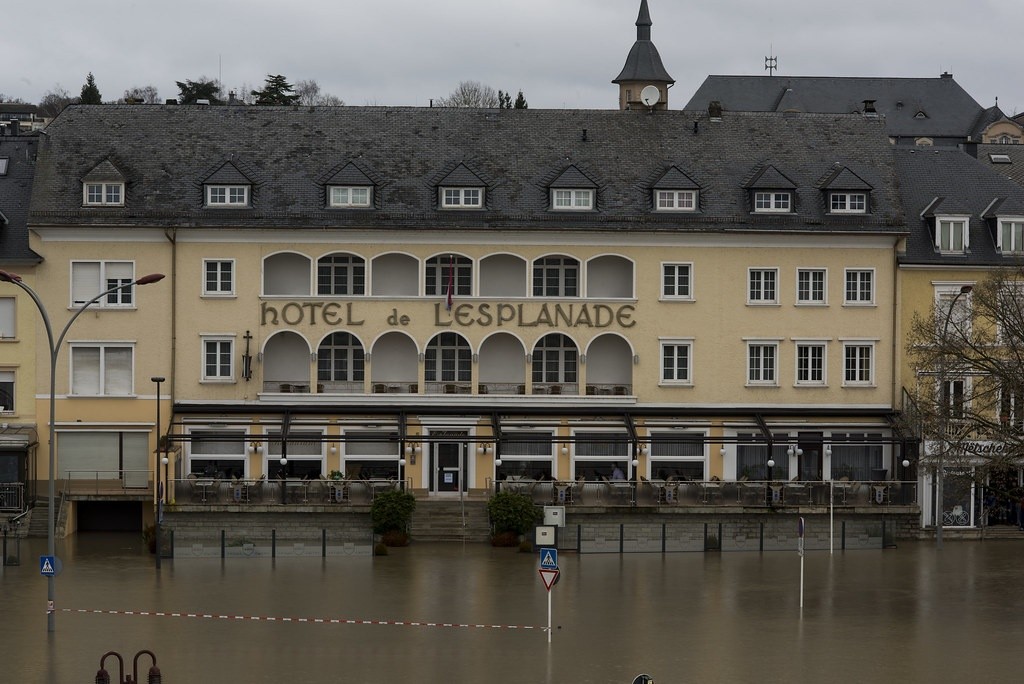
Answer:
[502,476,899,507]
[188,474,398,504]
[277,383,629,395]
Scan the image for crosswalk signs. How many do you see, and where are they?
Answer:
[540,548,557,568]
[40,556,55,574]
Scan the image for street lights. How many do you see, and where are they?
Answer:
[938,284,973,549]
[151,377,166,528]
[0,269,166,631]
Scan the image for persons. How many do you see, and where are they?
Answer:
[611,462,624,479]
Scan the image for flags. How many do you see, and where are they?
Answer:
[446,258,453,311]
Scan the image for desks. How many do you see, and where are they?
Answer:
[786,484,805,506]
[744,483,764,505]
[239,478,255,502]
[460,386,471,394]
[195,477,214,505]
[553,480,576,505]
[869,485,891,504]
[369,478,391,502]
[509,479,536,497]
[327,477,346,502]
[388,386,400,393]
[294,385,308,393]
[600,389,610,395]
[535,387,544,394]
[700,483,719,504]
[652,479,669,505]
[834,484,851,501]
[613,482,630,505]
[286,477,303,504]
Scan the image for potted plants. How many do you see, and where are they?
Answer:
[488,490,545,547]
[370,489,416,546]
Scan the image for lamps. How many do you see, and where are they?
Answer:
[719,444,727,455]
[330,443,337,454]
[902,460,910,467]
[580,353,586,363]
[478,442,502,466]
[632,443,648,466]
[257,352,263,362]
[768,445,832,467]
[527,353,531,363]
[473,353,478,363]
[561,443,568,455]
[248,442,264,453]
[420,353,425,363]
[366,353,370,362]
[280,454,288,465]
[311,352,317,361]
[634,354,639,363]
[399,442,421,466]
[162,453,169,465]
